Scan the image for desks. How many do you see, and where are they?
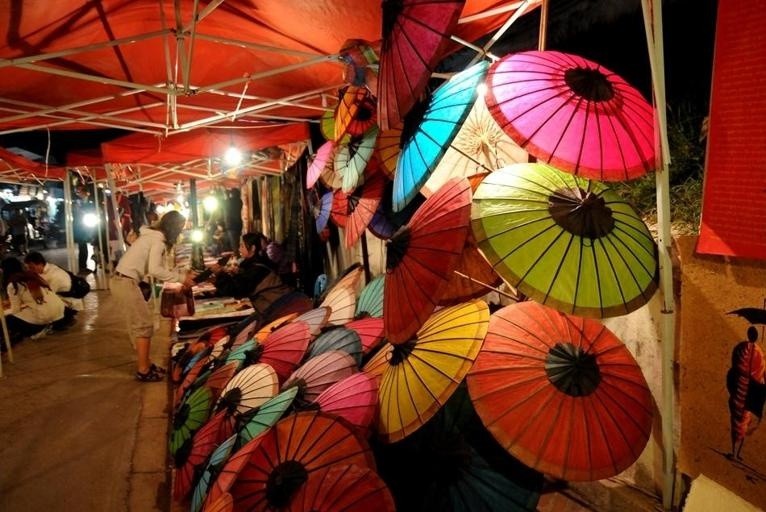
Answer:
[172,297,255,340]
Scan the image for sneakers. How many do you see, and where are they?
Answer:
[29,327,50,341]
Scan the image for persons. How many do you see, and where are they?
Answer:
[22,251,84,326]
[0,256,65,341]
[0,194,159,275]
[106,209,197,382]
[726,326,766,466]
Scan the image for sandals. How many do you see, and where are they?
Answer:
[136,364,166,383]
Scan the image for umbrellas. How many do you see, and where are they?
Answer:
[726,307,766,326]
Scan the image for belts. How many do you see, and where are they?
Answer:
[116,269,139,281]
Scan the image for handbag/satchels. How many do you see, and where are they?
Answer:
[57,271,90,299]
